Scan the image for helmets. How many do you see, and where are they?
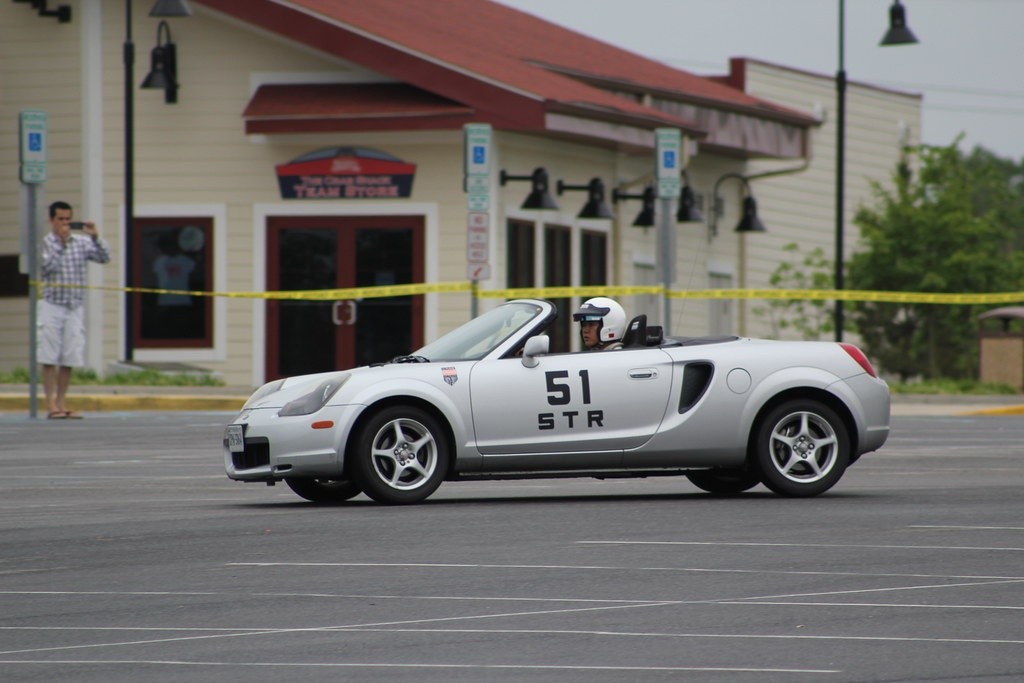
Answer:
[573,297,627,343]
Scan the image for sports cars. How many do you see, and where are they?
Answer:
[220,296,891,507]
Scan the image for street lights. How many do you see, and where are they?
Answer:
[125,1,195,363]
[835,1,924,345]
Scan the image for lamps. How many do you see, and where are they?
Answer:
[556,177,615,218]
[708,173,765,237]
[676,171,704,222]
[140,20,179,104]
[500,167,562,210]
[612,185,656,229]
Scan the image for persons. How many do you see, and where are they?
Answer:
[36,199,112,421]
[536,297,625,357]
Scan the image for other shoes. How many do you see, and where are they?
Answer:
[67,411,84,419]
[48,409,66,420]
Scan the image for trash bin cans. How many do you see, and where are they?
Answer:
[976,306,1024,389]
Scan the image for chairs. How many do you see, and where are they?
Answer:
[623,315,646,347]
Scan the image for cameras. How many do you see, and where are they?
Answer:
[67,222,84,230]
